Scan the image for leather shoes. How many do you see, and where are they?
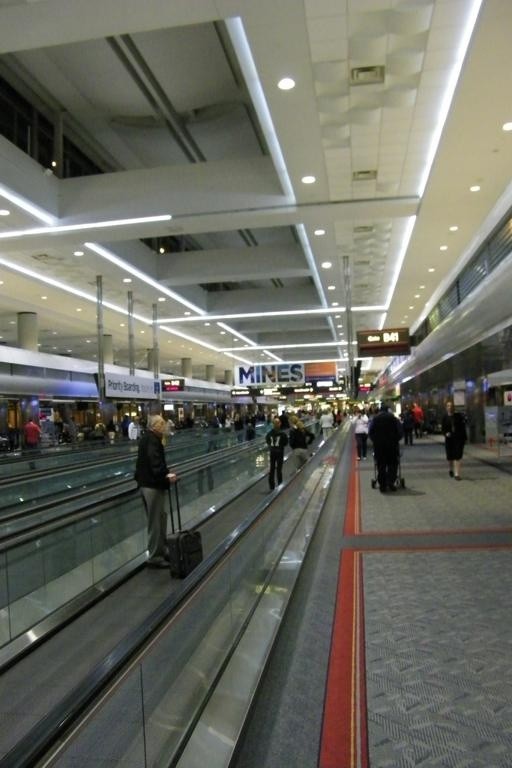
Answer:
[149,561,170,568]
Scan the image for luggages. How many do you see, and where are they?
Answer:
[163,477,205,579]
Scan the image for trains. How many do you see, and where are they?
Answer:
[0,359,280,452]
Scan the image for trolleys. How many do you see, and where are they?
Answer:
[370,449,405,490]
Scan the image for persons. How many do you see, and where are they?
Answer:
[161,411,258,453]
[259,398,468,495]
[134,416,177,570]
[1,413,134,452]
